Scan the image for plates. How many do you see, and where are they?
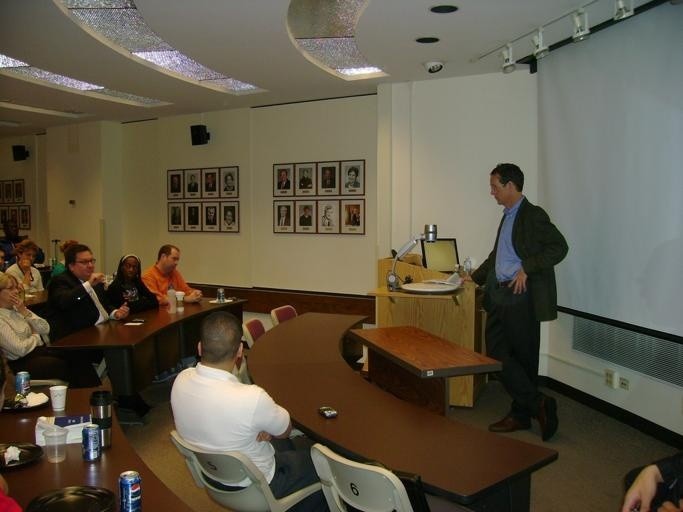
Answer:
[0,441,43,468]
[3,395,48,410]
[24,485,114,512]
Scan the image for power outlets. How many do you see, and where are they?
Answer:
[604,369,618,390]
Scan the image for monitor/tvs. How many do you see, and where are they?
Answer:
[421,237,460,273]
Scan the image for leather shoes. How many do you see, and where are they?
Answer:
[536,390,560,444]
[488,413,534,433]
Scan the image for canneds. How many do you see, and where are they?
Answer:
[15,371,31,394]
[216,288,225,303]
[117,470,143,512]
[81,424,102,462]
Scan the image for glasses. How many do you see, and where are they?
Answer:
[72,258,97,266]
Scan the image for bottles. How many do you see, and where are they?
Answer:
[218,289,225,302]
[166,285,176,314]
[463,256,471,273]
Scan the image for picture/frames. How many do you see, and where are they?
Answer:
[166,165,242,234]
[270,158,367,237]
[1,175,32,230]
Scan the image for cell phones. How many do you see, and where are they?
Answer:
[319,407,338,418]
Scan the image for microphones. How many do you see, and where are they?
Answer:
[12,145,29,161]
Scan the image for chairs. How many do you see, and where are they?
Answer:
[271,305,298,330]
[242,315,266,348]
[305,438,432,512]
[163,426,326,512]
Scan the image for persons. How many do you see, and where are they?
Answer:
[171,309,332,512]
[457,163,569,442]
[0,180,29,228]
[169,165,360,230]
[1,221,206,413]
[618,450,681,511]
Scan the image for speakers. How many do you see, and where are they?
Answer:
[191,125,210,145]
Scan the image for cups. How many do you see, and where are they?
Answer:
[42,429,69,463]
[88,389,115,449]
[48,385,67,412]
[175,291,184,312]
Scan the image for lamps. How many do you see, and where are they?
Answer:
[386,223,437,291]
[499,0,682,74]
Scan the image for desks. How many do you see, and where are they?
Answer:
[45,294,247,415]
[244,309,558,512]
[1,379,203,512]
[341,319,509,423]
